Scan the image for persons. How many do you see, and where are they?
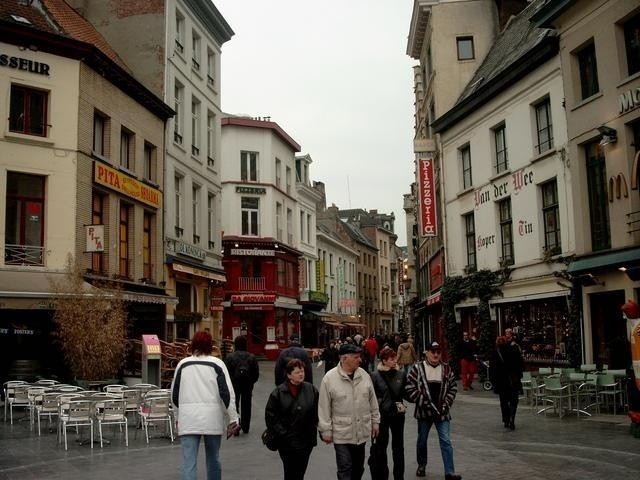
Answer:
[621,299,640,424]
[276,327,524,431]
[316,343,382,480]
[368,346,422,480]
[404,341,461,480]
[223,334,260,433]
[265,359,319,480]
[171,330,242,480]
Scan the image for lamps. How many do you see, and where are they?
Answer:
[597,126,618,148]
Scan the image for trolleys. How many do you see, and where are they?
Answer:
[475,354,492,390]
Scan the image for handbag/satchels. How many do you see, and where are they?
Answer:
[394,402,406,413]
[262,430,278,451]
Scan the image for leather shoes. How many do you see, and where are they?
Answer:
[416,465,425,476]
[445,474,462,480]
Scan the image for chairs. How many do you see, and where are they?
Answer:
[521,364,626,418]
[3,379,176,450]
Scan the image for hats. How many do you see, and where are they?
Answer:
[289,334,299,341]
[427,342,440,349]
[338,344,363,354]
